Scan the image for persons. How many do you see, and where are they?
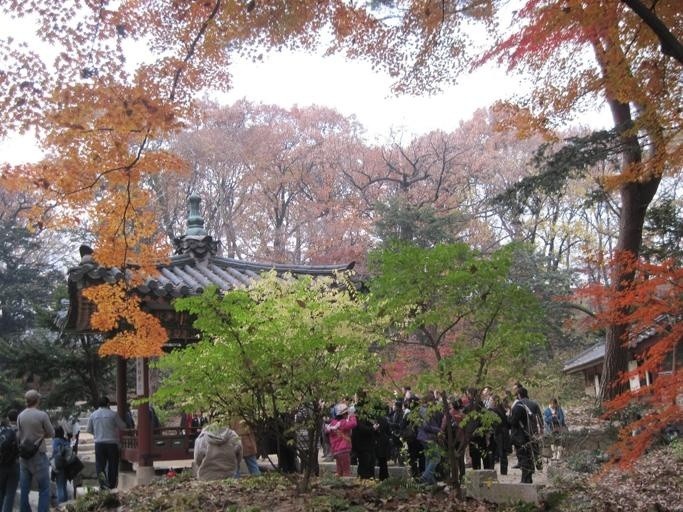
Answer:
[0,390,135,512]
[150,377,568,480]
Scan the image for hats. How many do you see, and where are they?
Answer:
[334,403,349,416]
[25,390,42,405]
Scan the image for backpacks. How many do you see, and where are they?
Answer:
[1,426,18,462]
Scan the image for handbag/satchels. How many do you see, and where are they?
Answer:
[50,447,74,472]
[18,440,38,459]
[527,413,543,442]
